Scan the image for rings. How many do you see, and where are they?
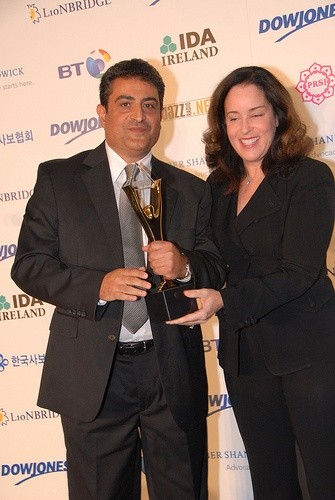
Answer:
[188,325,195,329]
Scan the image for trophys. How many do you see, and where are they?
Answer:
[122,161,200,329]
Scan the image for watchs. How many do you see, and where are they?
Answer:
[177,253,193,282]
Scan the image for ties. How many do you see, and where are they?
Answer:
[118,164,149,334]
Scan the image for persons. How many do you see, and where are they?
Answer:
[164,65,335,500]
[11,59,224,500]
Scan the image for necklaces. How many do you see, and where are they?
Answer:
[243,175,265,185]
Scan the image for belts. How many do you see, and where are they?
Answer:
[115,339,155,356]
